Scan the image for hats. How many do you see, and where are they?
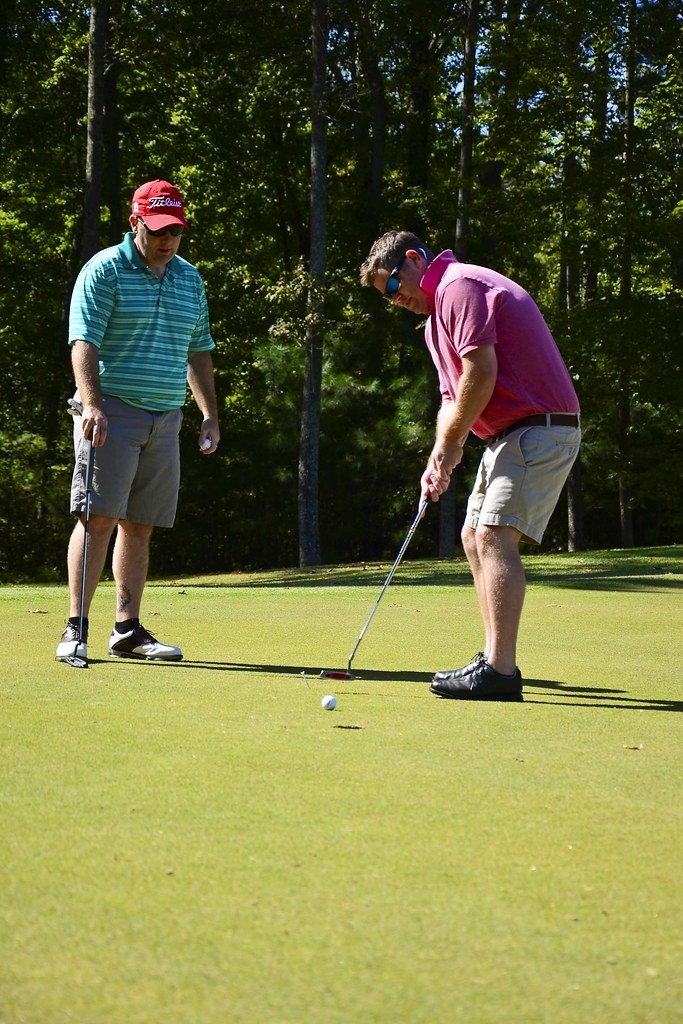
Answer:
[132,179,190,232]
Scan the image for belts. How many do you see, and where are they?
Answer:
[488,414,579,446]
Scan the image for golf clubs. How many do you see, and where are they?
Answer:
[63,426,89,668]
[319,493,429,680]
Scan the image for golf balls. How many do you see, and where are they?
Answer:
[321,695,337,710]
[200,439,211,450]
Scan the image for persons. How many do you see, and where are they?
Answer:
[56,179,223,665]
[360,229,581,703]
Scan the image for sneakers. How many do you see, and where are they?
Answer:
[430,655,524,703]
[55,624,87,662]
[108,628,184,661]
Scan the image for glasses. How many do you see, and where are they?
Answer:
[381,253,408,304]
[136,217,184,237]
